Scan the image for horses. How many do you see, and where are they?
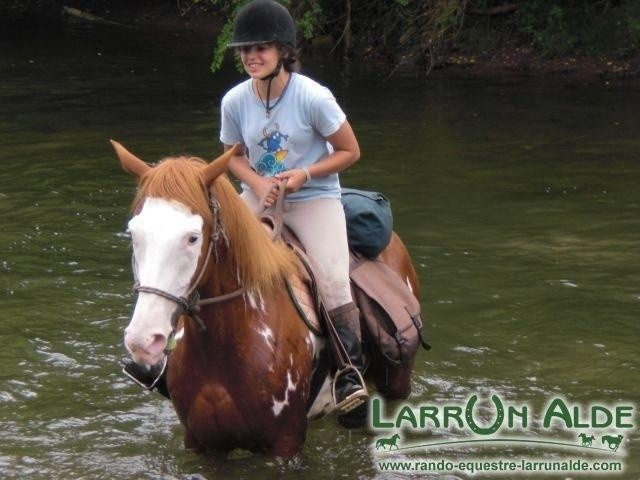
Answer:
[109,139,423,459]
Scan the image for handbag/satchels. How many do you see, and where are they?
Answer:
[341,188,393,261]
[349,261,424,367]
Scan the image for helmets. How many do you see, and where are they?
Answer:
[226,0,297,48]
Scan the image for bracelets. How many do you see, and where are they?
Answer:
[303,167,311,184]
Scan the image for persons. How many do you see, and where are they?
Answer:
[126,0,368,429]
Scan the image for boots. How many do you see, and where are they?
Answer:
[328,302,366,404]
[125,330,176,400]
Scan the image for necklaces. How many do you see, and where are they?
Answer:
[256,72,291,119]
